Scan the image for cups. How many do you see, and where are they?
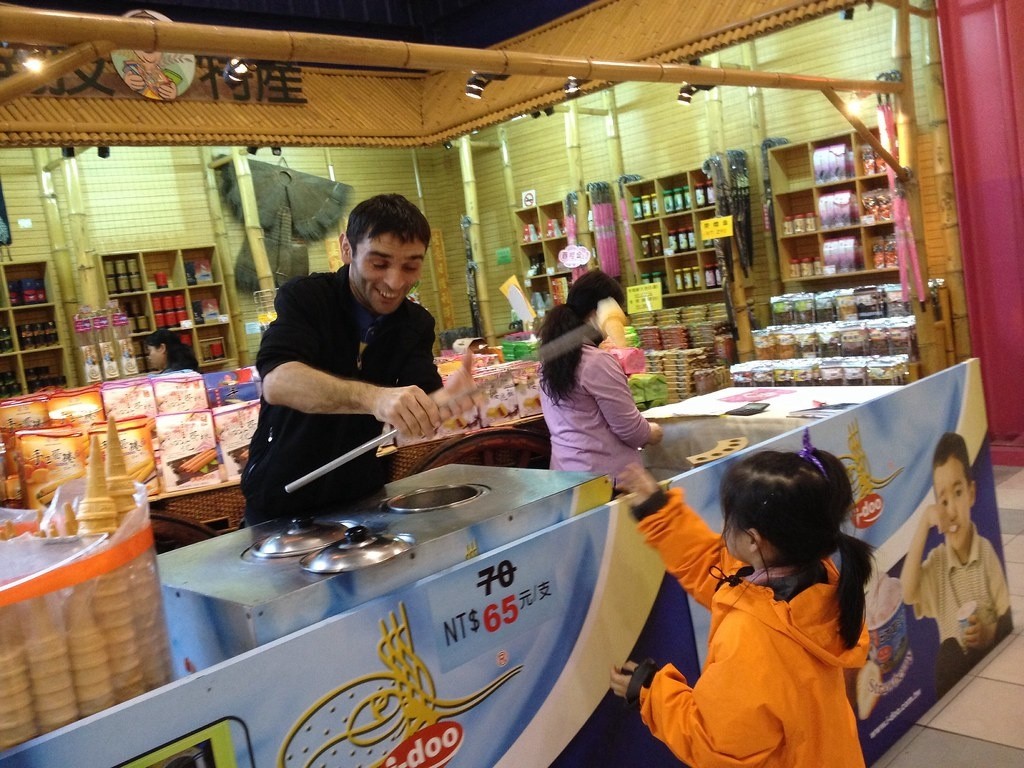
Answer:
[867,577,908,683]
[957,601,978,645]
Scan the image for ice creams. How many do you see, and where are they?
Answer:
[955,601,978,636]
[861,568,909,673]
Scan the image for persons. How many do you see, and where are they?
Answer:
[610,448,874,768]
[900,433,1013,700]
[538,268,664,501]
[240,193,490,528]
[145,329,200,374]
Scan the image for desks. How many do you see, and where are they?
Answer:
[642,385,903,480]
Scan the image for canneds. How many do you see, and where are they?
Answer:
[104,259,225,363]
[631,179,724,295]
[783,212,820,277]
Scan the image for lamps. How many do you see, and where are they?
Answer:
[678,82,715,105]
[443,141,452,149]
[839,7,855,20]
[464,71,510,99]
[222,58,257,88]
[564,76,581,98]
[544,106,555,115]
[97,146,110,158]
[246,146,258,155]
[271,146,281,155]
[62,145,75,157]
[531,111,541,119]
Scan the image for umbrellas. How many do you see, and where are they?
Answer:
[876,69,926,313]
[565,174,645,287]
[460,216,482,337]
[703,138,789,282]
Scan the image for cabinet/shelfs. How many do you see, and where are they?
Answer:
[93,243,241,374]
[512,192,603,319]
[0,255,79,398]
[732,282,944,386]
[767,125,910,293]
[621,159,753,308]
